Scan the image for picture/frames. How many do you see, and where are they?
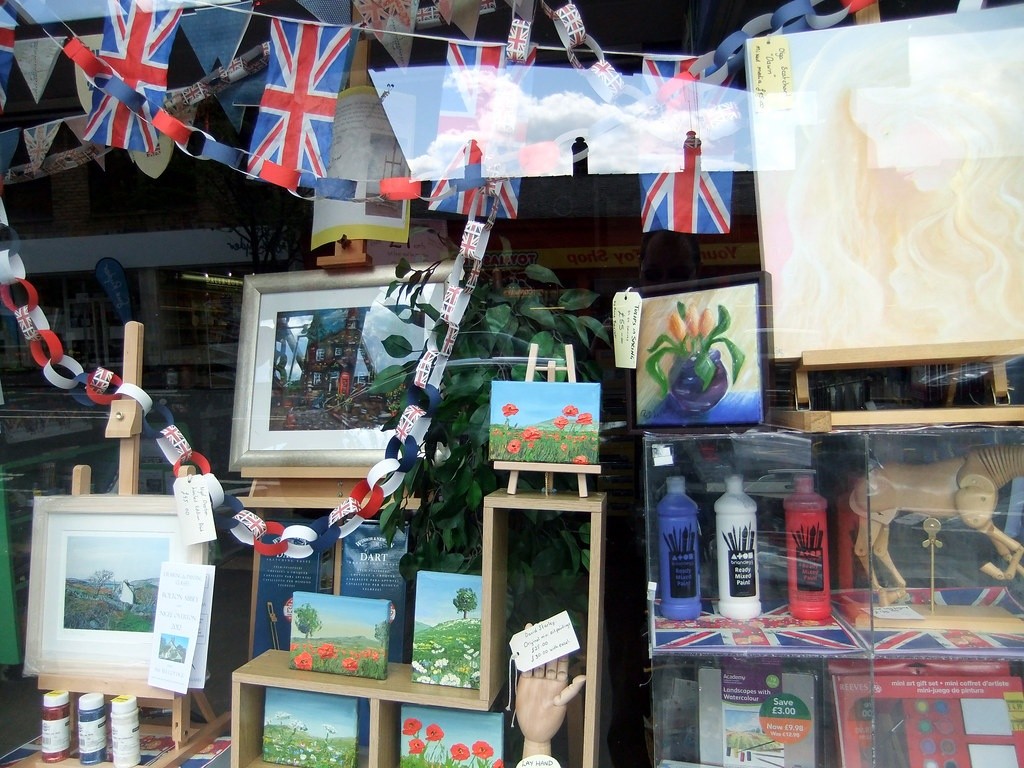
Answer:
[23,495,208,690]
[229,258,461,472]
[628,271,774,432]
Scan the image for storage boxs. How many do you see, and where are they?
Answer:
[825,660,1024,767]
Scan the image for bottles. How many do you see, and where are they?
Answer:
[656,476,702,622]
[784,472,829,620]
[79,693,110,765]
[38,690,70,763]
[112,692,142,765]
[713,475,762,619]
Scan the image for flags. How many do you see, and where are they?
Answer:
[82,0,186,158]
[246,18,351,189]
[638,58,734,236]
[427,43,537,220]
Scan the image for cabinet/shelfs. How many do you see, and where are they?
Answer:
[0,265,248,575]
[230,489,609,768]
[639,426,1024,767]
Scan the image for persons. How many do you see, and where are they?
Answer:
[514,623,587,759]
